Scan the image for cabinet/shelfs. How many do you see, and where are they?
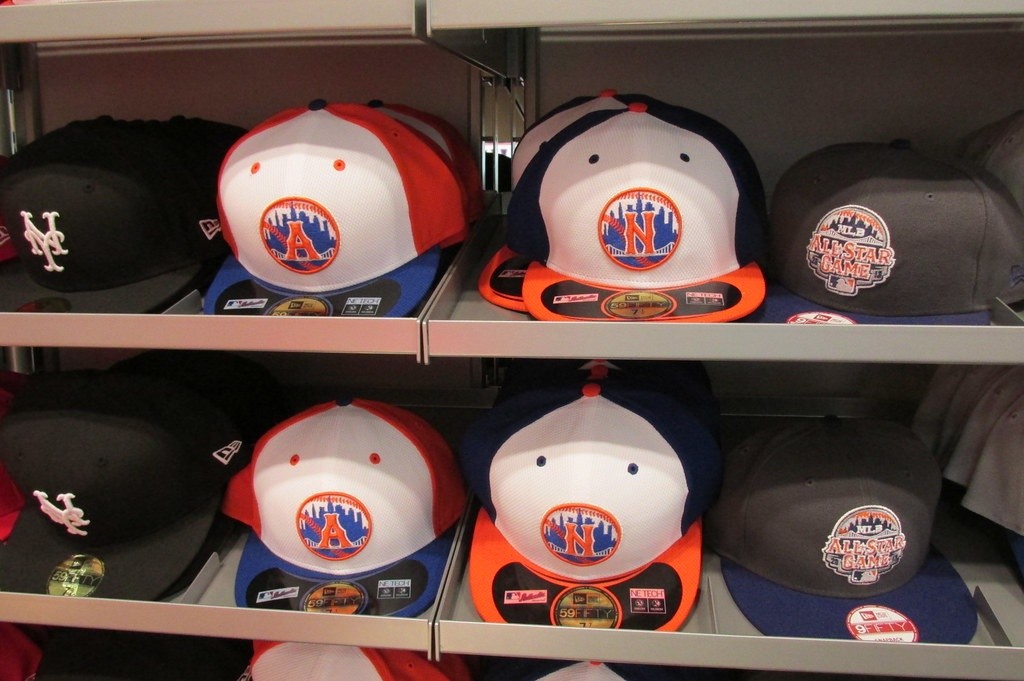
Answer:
[0,0,1024,680]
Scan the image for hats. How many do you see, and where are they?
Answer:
[0,98,484,318]
[480,86,1024,327]
[0,346,978,681]
